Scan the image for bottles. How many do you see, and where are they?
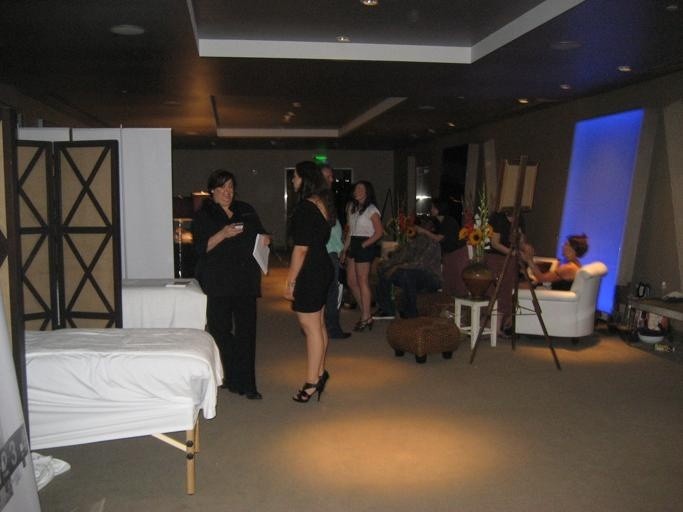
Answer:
[660,281,670,301]
[636,281,649,297]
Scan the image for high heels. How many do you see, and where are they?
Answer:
[351,316,374,333]
[291,368,330,404]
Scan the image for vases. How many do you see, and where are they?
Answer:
[395,231,408,246]
[461,261,497,300]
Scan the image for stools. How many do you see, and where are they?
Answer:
[452,294,499,348]
[386,316,460,360]
[394,288,449,316]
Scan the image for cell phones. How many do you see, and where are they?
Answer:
[235,222,244,229]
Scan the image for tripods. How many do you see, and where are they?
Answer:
[469,228,562,371]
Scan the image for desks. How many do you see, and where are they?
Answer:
[624,296,683,362]
[173,238,195,278]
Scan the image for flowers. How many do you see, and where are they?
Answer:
[456,182,495,265]
[389,208,416,246]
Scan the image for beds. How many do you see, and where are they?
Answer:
[24,322,227,498]
[120,274,209,330]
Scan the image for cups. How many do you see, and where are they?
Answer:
[235,222,245,230]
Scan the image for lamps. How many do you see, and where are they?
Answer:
[172,194,195,278]
[192,191,211,214]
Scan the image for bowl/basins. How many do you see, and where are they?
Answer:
[639,332,665,345]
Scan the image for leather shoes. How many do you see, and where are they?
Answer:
[328,331,351,339]
[229,387,263,400]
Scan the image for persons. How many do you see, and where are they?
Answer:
[339,180,381,333]
[283,160,334,403]
[189,169,274,400]
[321,164,351,340]
[371,197,588,319]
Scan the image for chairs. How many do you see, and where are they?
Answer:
[509,260,608,346]
[530,256,560,279]
